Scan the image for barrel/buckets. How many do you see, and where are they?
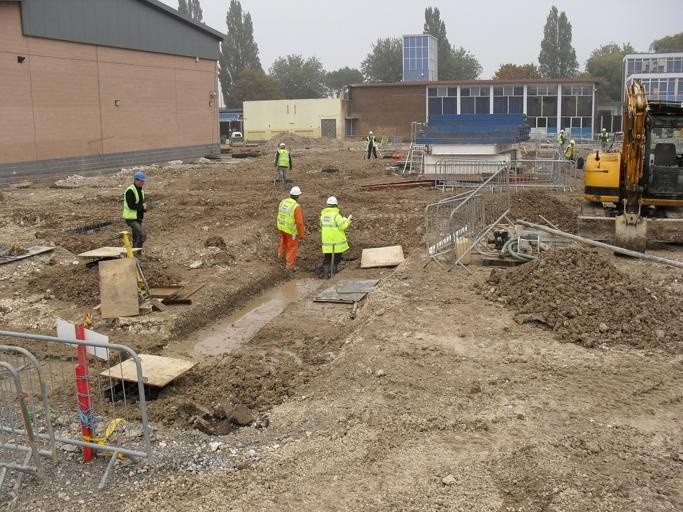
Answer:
[455,236,472,265]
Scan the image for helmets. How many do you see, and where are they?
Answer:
[280,143,284,147]
[369,131,372,134]
[134,172,146,181]
[290,185,302,195]
[327,196,337,205]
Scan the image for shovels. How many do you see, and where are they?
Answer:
[136,259,167,311]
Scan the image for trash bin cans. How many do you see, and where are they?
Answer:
[230,132,242,141]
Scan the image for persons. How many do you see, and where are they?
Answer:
[275,143,292,188]
[319,196,352,279]
[599,128,609,152]
[563,140,576,160]
[366,131,377,159]
[559,130,565,145]
[276,186,304,272]
[122,172,147,255]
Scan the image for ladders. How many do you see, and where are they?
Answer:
[409,150,423,175]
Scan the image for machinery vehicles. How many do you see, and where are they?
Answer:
[577,78,683,256]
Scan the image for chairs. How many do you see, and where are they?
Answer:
[654,143,679,188]
